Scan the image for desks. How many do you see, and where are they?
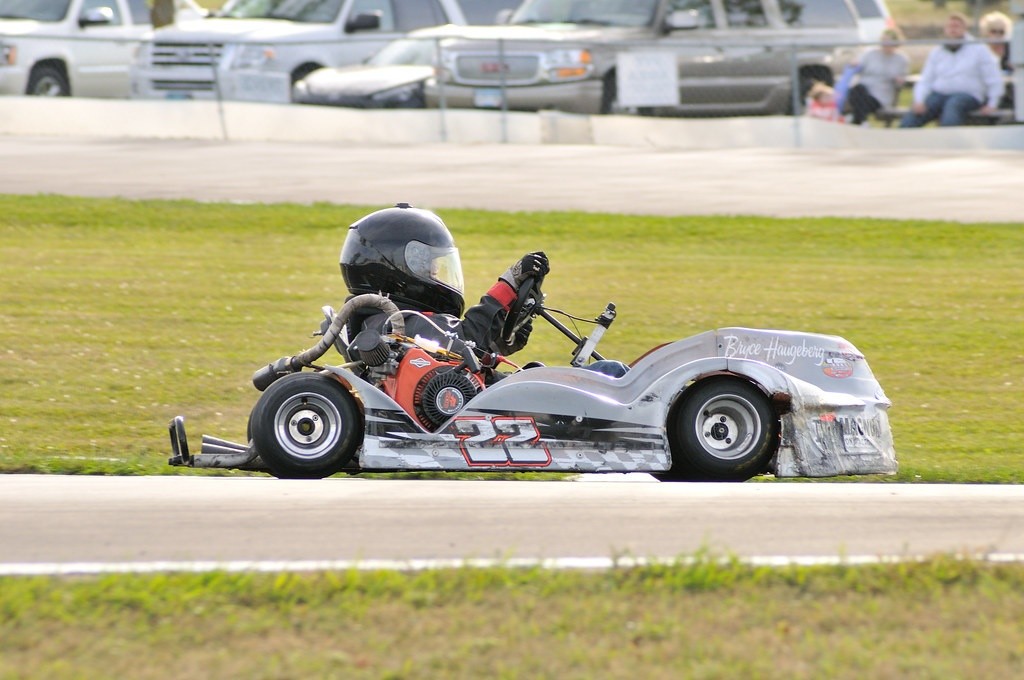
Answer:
[907,70,1012,88]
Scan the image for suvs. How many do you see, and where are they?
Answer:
[420,1,896,120]
[130,0,525,106]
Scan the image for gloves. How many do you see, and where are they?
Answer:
[498,252,550,293]
[488,315,534,357]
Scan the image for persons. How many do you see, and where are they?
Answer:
[340,203,629,378]
[977,12,1014,124]
[834,28,909,124]
[808,83,845,122]
[900,13,1002,127]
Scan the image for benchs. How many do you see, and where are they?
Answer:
[874,106,1011,126]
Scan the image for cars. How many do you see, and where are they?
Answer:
[1,0,213,97]
[291,25,449,109]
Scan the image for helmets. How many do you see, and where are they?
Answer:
[340,202,466,320]
